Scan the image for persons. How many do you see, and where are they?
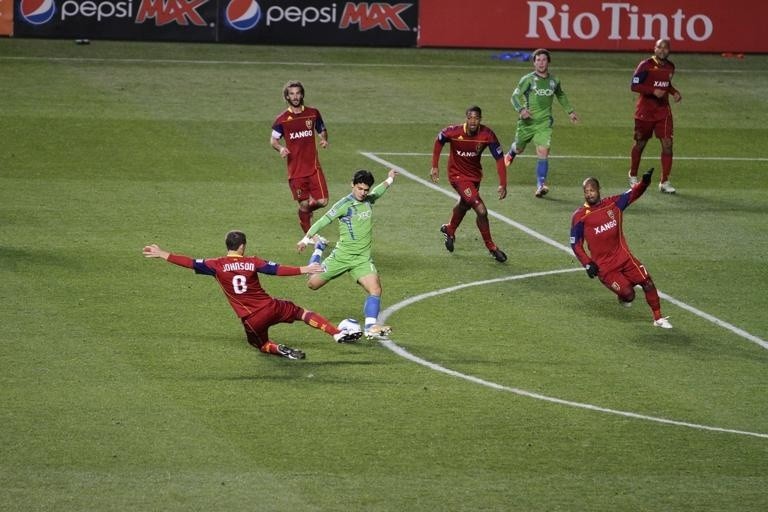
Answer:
[569,166,674,329]
[270,81,329,243]
[297,168,398,339]
[502,49,578,196]
[143,231,363,360]
[628,39,682,194]
[430,106,508,262]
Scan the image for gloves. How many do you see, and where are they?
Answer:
[642,166,654,186]
[586,262,600,279]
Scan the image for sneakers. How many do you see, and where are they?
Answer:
[333,329,362,343]
[439,222,456,252]
[628,169,640,188]
[311,233,330,251]
[653,317,673,329]
[489,246,507,262]
[534,184,549,198]
[363,325,392,340]
[503,152,513,167]
[615,295,632,307]
[277,343,306,360]
[658,180,677,194]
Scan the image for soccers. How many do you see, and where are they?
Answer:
[227,0,262,30]
[338,318,362,343]
[21,1,54,24]
